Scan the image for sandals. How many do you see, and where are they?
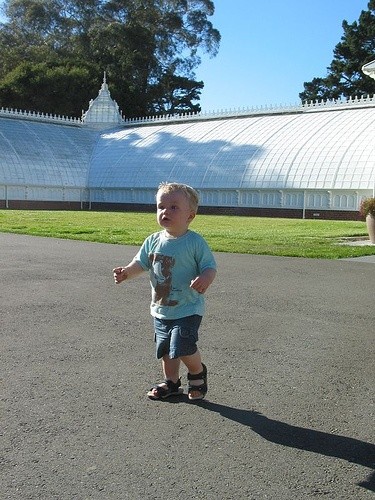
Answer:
[187,362,208,402]
[147,376,184,400]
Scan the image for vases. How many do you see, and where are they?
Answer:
[366,213,375,244]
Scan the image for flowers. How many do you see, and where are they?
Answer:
[359,197,375,216]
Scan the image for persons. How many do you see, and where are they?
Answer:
[111,180,217,402]
